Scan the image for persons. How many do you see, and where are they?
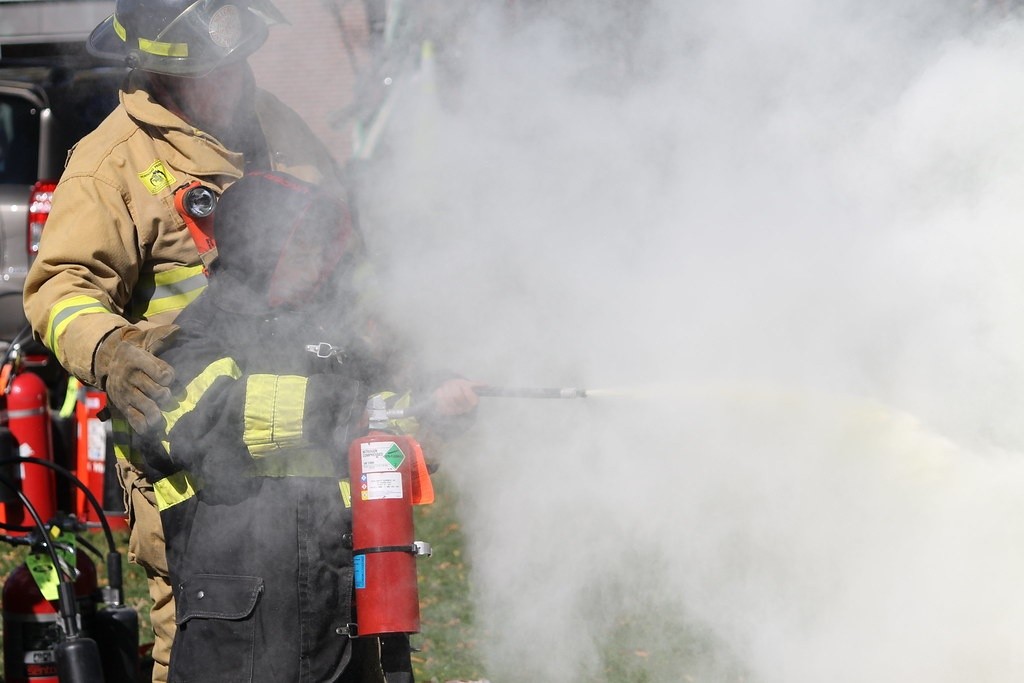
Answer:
[126,173,417,682]
[23,0,476,683]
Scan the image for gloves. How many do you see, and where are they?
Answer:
[94,324,182,436]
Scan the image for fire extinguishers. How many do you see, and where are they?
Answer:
[0,331,57,537]
[84,391,129,530]
[348,383,587,637]
[2,516,104,683]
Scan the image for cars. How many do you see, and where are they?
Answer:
[1,0,364,402]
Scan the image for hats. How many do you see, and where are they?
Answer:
[213,170,352,311]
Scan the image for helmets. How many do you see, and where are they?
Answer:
[85,0,270,79]
